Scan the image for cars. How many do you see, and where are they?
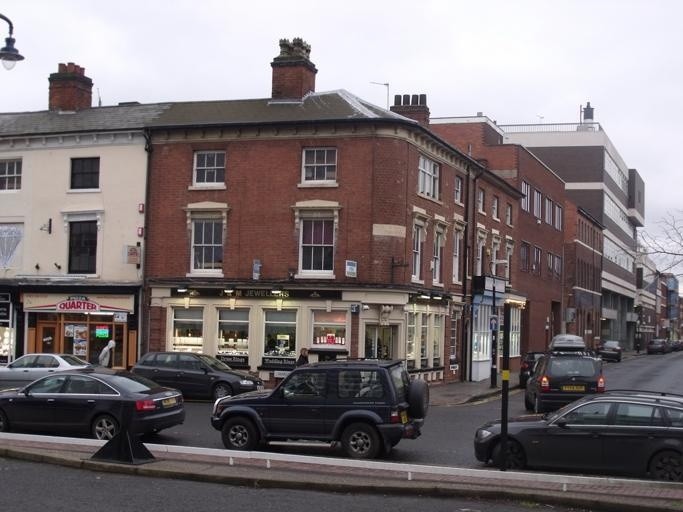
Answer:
[646,339,683,355]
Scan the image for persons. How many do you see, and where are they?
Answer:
[267,336,276,352]
[297,348,310,366]
[98,340,117,368]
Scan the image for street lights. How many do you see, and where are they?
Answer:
[488,256,511,391]
[635,318,641,355]
[0,13,26,70]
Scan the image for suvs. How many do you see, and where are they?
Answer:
[473,334,683,483]
